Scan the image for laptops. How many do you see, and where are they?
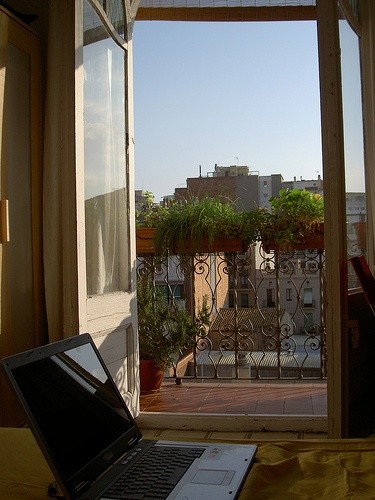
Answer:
[1,333,258,500]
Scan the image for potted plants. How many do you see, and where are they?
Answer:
[135,190,169,255]
[132,270,212,395]
[151,173,263,272]
[258,187,324,254]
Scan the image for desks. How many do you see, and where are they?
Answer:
[0,427,375,499]
